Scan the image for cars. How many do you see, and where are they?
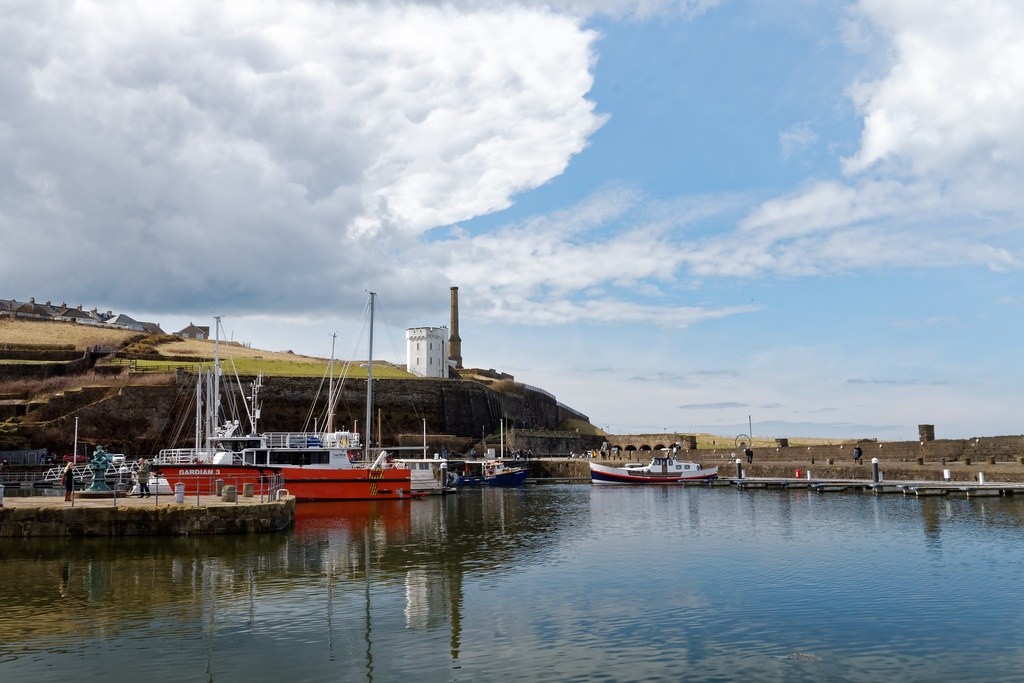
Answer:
[112,454,126,465]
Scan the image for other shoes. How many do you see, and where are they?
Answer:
[65,498,72,501]
[138,496,143,498]
[147,495,151,498]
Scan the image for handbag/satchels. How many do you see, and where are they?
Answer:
[61,474,65,486]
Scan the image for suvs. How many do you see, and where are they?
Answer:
[62,453,91,464]
[102,454,113,463]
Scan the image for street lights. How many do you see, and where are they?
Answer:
[423,419,427,459]
[499,419,504,459]
[73,416,79,464]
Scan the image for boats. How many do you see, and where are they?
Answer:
[449,461,529,487]
[589,448,719,484]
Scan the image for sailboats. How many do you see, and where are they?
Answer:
[33,287,458,499]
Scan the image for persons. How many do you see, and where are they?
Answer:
[442,447,448,460]
[470,448,477,461]
[745,449,753,464]
[512,449,533,461]
[91,446,107,464]
[584,450,588,457]
[136,457,151,498]
[592,450,609,460]
[60,462,73,501]
[853,448,863,463]
